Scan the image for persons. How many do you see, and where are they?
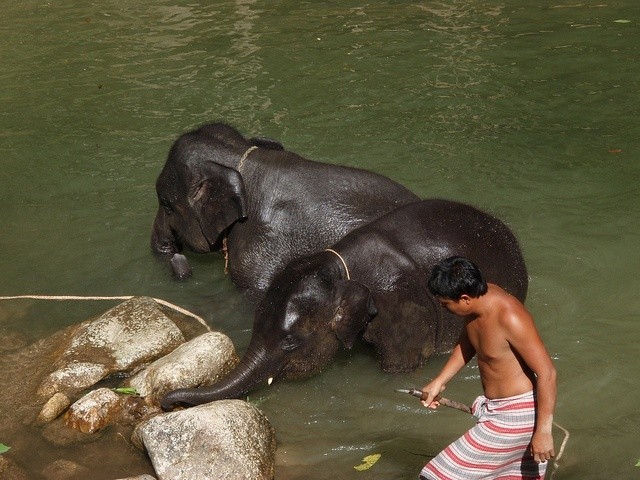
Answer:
[418,256,558,480]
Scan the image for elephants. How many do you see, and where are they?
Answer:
[162,198,528,407]
[149,123,420,308]
